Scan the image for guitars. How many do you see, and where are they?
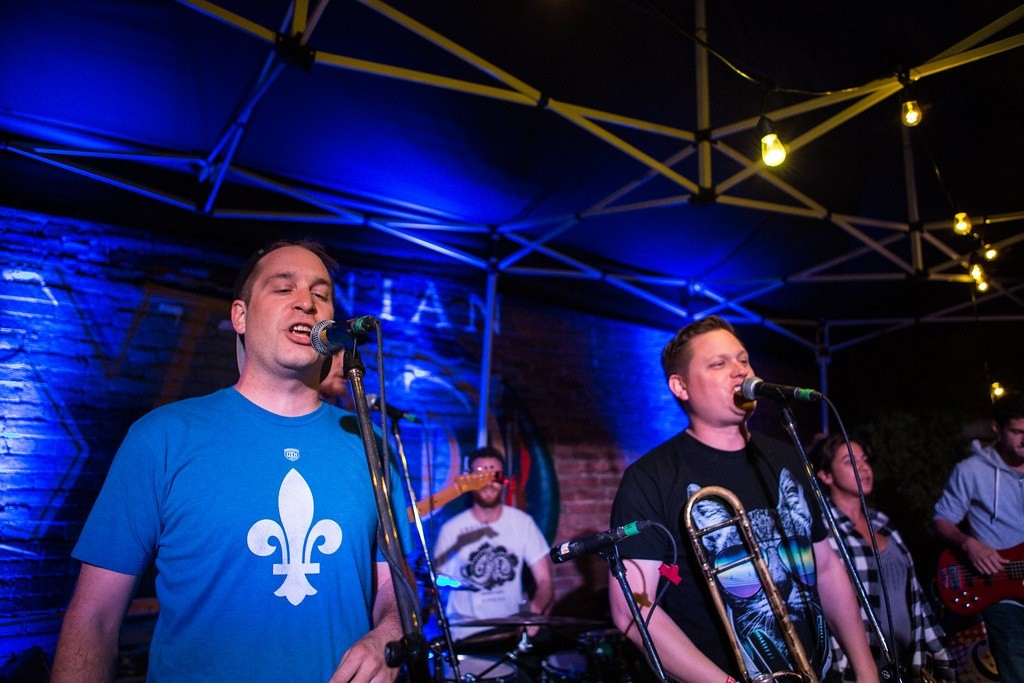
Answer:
[406,472,508,523]
[936,542,1024,618]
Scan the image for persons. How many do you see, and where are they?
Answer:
[51,241,418,683]
[432,447,554,644]
[932,391,1024,683]
[610,315,956,683]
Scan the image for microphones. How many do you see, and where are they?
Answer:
[365,394,423,425]
[310,316,381,357]
[549,520,653,564]
[740,376,823,404]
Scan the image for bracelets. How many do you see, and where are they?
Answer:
[725,674,737,683]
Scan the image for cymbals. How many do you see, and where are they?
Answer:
[455,627,522,646]
[449,612,608,626]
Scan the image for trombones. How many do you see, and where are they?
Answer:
[684,486,822,683]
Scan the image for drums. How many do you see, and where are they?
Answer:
[539,650,590,683]
[403,652,520,683]
[578,627,659,683]
